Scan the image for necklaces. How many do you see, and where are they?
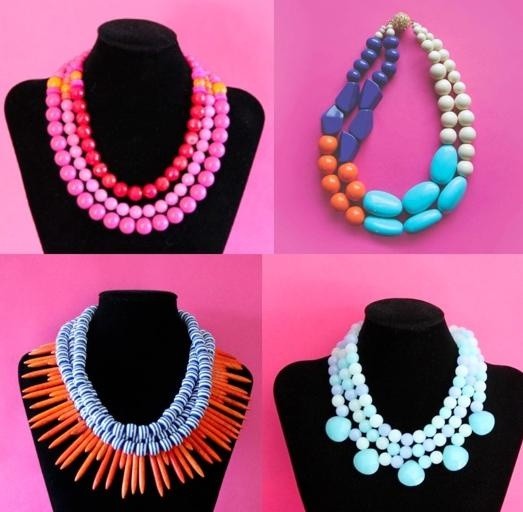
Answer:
[16,295,253,505]
[313,12,478,240]
[44,38,231,237]
[322,315,496,487]
